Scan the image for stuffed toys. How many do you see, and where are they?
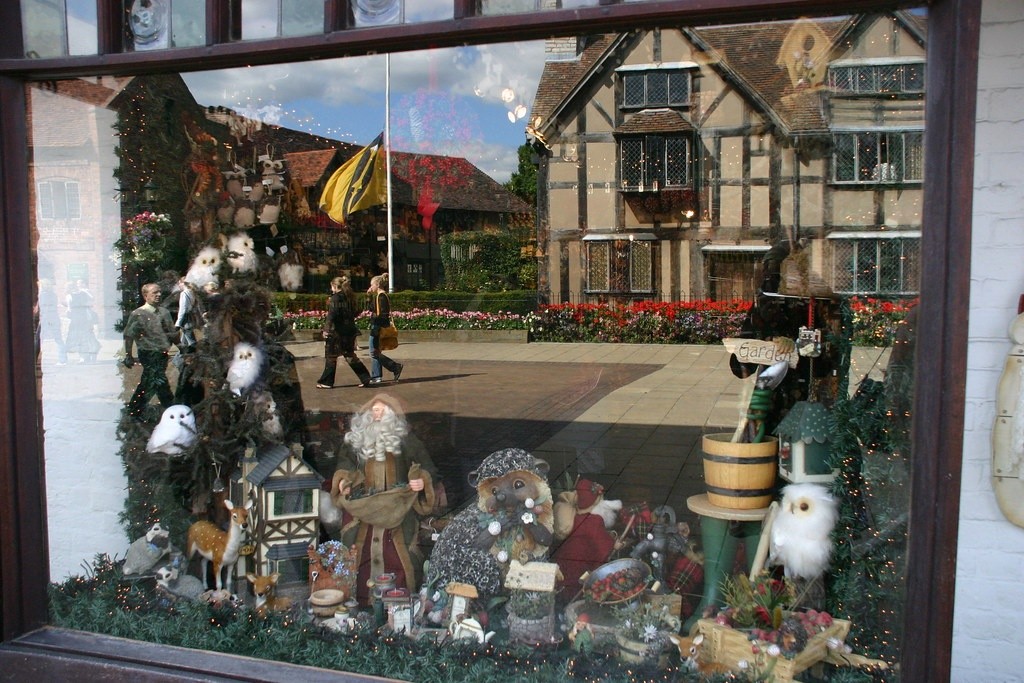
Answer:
[470,448,556,571]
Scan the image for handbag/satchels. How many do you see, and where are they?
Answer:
[379,321,398,352]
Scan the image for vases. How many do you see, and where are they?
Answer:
[508,612,555,642]
[697,611,851,683]
[615,631,669,670]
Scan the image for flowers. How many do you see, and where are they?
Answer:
[683,557,851,683]
[609,592,679,644]
[507,584,564,619]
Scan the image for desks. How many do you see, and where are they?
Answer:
[685,494,773,633]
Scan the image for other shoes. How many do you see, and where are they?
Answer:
[369,377,382,382]
[358,379,370,388]
[316,384,332,388]
[394,363,403,380]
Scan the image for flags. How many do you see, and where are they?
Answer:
[317,131,387,226]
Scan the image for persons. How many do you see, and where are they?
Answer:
[551,479,624,607]
[172,278,206,405]
[724,239,851,453]
[120,282,186,421]
[314,276,376,388]
[357,273,404,386]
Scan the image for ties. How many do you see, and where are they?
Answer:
[155,309,175,343]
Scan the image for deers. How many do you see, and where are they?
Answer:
[186,498,253,593]
[668,632,733,683]
[244,572,296,619]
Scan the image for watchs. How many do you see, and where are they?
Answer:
[126,352,132,356]
